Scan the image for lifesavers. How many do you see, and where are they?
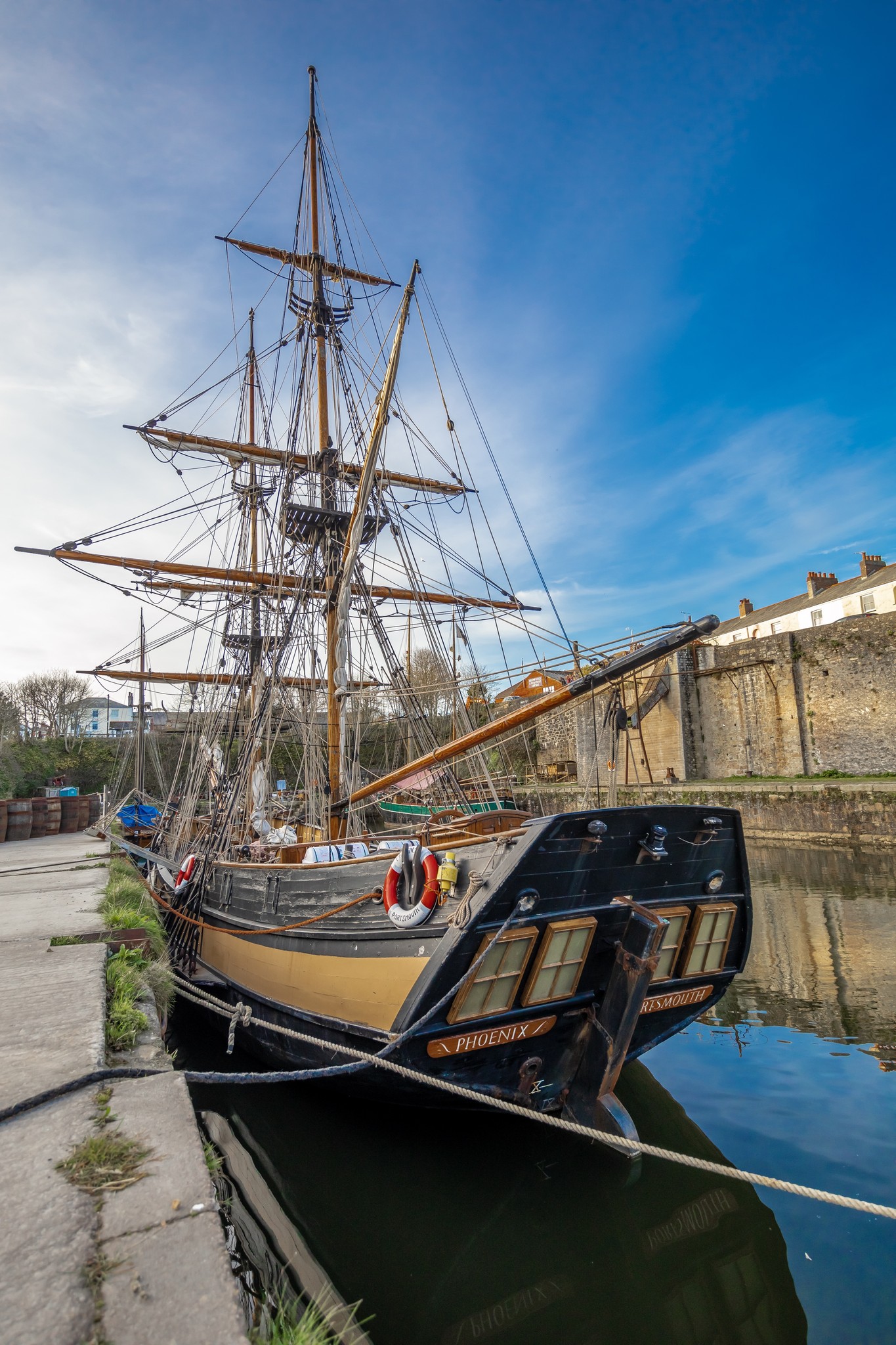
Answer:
[383,844,439,929]
[174,853,196,894]
[607,760,615,770]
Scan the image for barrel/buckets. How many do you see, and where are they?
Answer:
[458,778,517,790]
[0,794,101,843]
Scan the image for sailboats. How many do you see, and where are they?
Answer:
[14,59,755,1120]
